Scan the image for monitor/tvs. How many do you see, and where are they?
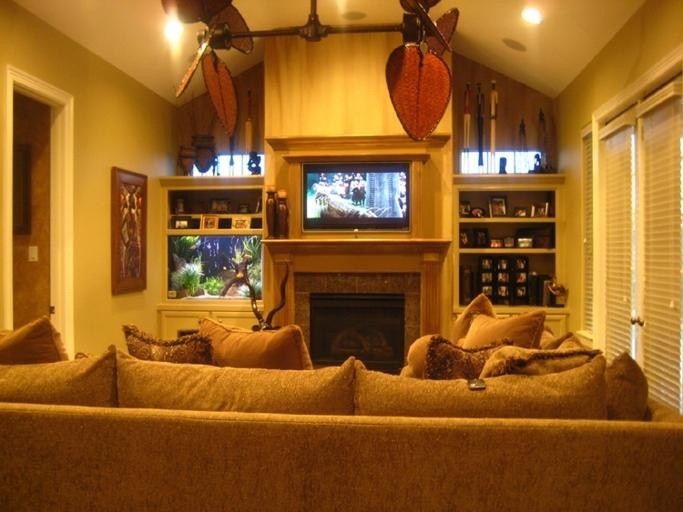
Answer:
[301,163,409,231]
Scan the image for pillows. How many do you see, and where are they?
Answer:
[398,294,604,379]
[1,315,69,365]
[121,322,212,366]
[354,352,648,422]
[107,343,356,415]
[196,313,315,370]
[1,342,119,409]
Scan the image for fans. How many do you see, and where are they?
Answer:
[160,0,459,142]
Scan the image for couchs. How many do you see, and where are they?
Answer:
[0,397,683,512]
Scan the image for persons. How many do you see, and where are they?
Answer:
[320,172,366,207]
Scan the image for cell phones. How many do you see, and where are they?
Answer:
[469,378,485,390]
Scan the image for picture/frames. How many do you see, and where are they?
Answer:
[488,195,549,218]
[170,198,252,231]
[14,144,31,235]
[110,165,148,296]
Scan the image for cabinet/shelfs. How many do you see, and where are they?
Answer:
[452,172,566,315]
[158,176,263,305]
